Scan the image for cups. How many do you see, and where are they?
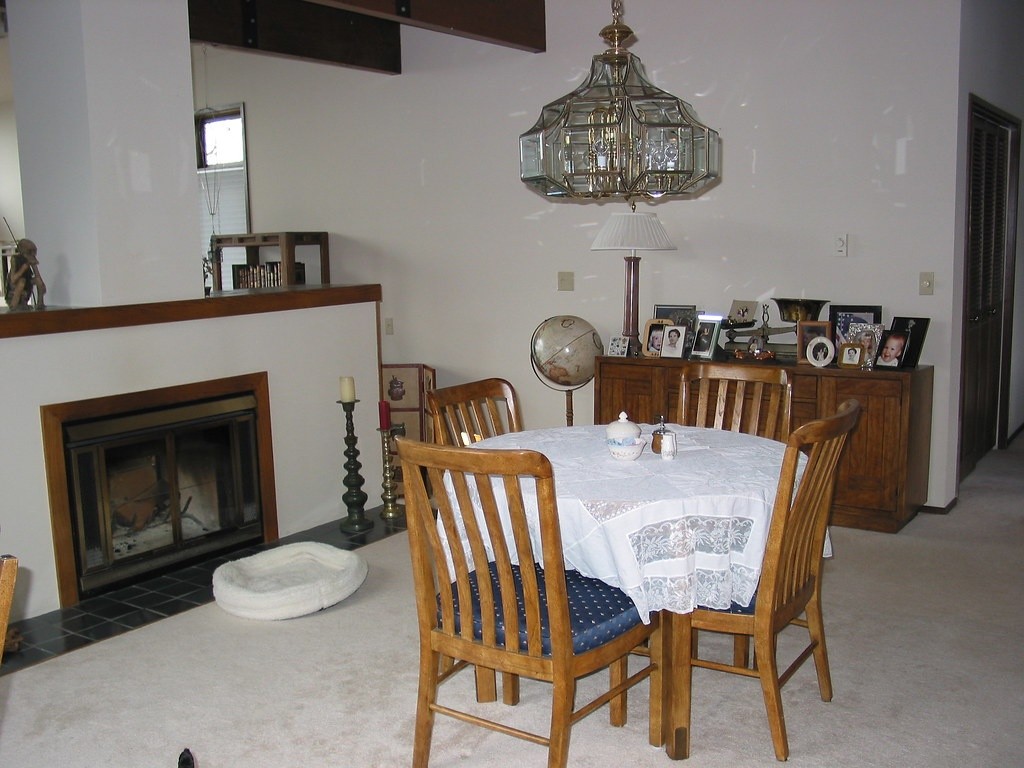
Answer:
[661,433,677,459]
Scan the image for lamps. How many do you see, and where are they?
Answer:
[519,0,719,202]
[590,201,677,357]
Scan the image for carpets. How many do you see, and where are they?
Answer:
[0,428,1024,768]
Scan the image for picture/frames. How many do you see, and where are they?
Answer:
[891,317,930,369]
[660,325,687,359]
[728,299,759,324]
[848,323,885,367]
[806,335,835,368]
[654,305,696,332]
[797,321,831,364]
[837,343,865,369]
[828,305,882,363]
[642,319,674,357]
[873,330,910,367]
[691,315,723,361]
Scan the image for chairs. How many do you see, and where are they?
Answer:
[395,364,860,768]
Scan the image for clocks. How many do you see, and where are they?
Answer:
[608,336,630,357]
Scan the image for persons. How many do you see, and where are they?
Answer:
[648,330,662,351]
[861,334,871,361]
[846,348,857,363]
[876,334,906,367]
[7,239,47,310]
[816,348,824,361]
[668,329,681,347]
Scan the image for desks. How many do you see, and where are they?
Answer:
[437,423,832,746]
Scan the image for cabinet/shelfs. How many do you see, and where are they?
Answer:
[211,231,330,291]
[594,355,934,534]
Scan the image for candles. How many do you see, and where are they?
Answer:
[340,376,355,402]
[379,401,390,430]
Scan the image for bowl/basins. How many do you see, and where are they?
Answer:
[607,436,646,461]
[605,411,642,438]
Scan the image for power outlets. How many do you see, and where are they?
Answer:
[385,318,393,335]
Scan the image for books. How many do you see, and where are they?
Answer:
[239,261,305,288]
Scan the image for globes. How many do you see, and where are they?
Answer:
[530,315,604,428]
